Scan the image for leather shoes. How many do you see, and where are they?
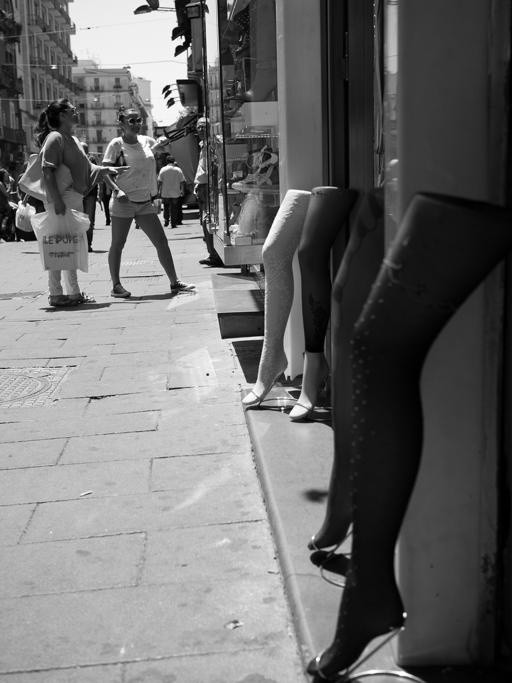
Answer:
[199,256,223,266]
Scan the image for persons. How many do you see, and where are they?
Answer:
[276,180,339,421]
[237,185,313,407]
[99,104,197,298]
[288,185,510,681]
[34,96,133,310]
[1,138,188,253]
[192,117,221,268]
[295,178,394,561]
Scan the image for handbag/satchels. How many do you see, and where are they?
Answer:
[16,131,90,272]
[110,138,126,176]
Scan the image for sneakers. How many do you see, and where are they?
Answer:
[171,280,195,293]
[48,292,95,306]
[111,283,130,296]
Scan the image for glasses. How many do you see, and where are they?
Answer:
[65,107,76,114]
[129,118,143,125]
